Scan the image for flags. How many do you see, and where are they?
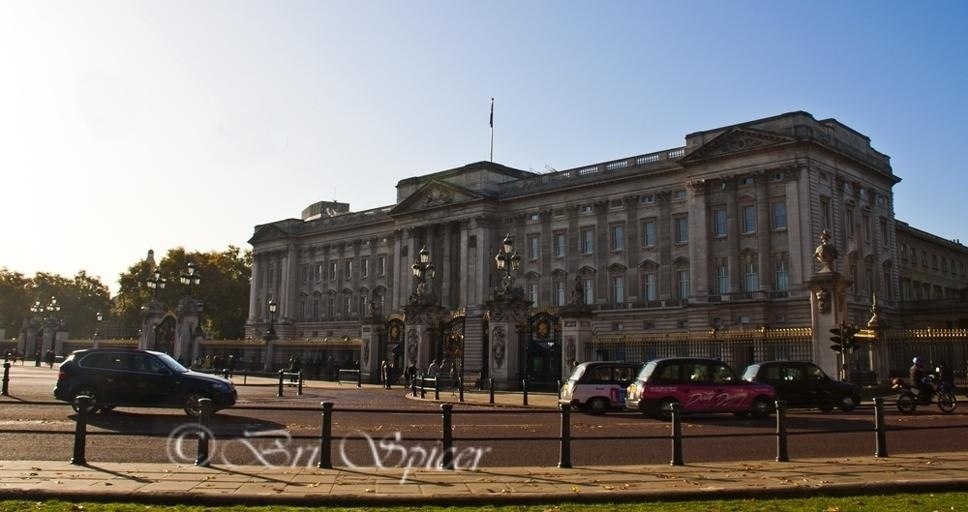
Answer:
[489,97,494,129]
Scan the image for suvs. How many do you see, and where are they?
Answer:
[743,358,862,412]
[53,345,238,420]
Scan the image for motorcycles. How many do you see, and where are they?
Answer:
[891,367,958,414]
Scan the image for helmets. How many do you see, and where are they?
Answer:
[912,356,922,365]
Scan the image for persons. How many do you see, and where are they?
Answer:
[910,356,936,406]
[277,352,459,391]
[166,350,243,378]
[938,360,956,394]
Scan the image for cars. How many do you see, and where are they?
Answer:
[556,360,646,414]
[622,357,779,423]
